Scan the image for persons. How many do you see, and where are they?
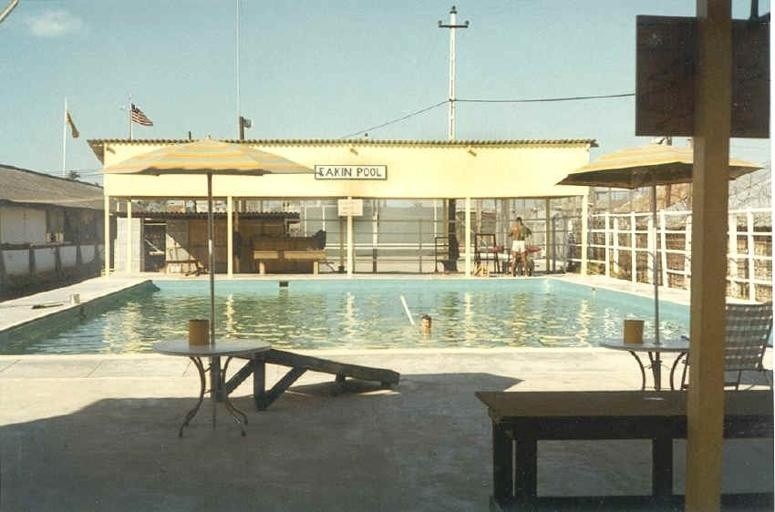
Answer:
[422,315,435,329]
[505,215,531,277]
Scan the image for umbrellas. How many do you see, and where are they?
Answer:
[555,142,766,345]
[83,134,324,345]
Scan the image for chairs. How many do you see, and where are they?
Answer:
[722,298,773,389]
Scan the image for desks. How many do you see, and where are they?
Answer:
[154,335,271,439]
[606,336,693,393]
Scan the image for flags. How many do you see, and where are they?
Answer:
[131,104,154,127]
[66,113,80,139]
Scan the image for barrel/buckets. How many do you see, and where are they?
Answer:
[70,293,81,304]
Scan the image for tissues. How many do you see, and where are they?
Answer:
[623,311,644,344]
[188,314,210,344]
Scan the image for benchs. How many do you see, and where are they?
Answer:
[472,389,773,512]
